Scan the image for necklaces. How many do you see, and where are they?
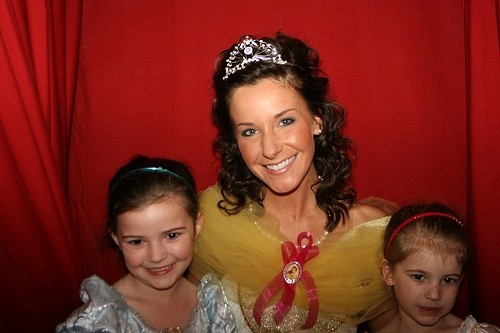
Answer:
[249,201,331,248]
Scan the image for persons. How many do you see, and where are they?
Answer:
[55,154,239,333]
[378,203,500,333]
[183,32,398,333]
